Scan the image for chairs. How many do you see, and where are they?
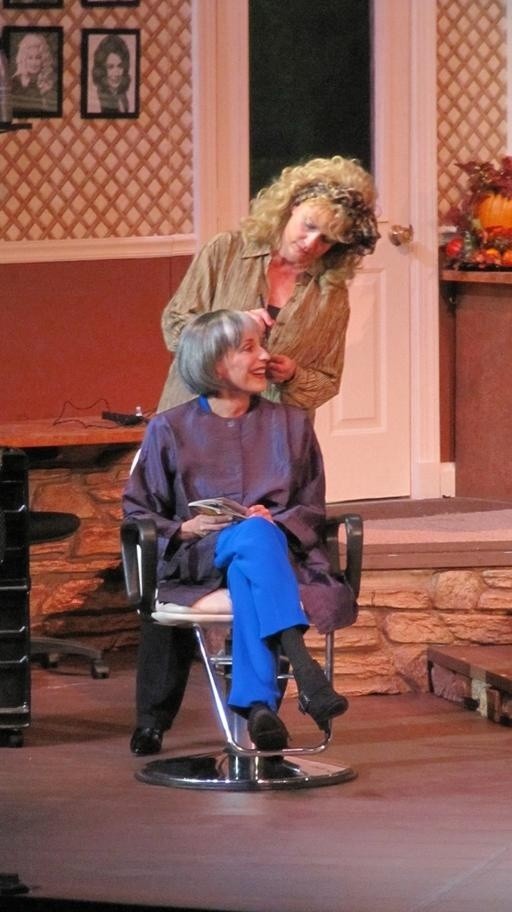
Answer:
[120,449,364,792]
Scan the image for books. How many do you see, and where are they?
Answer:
[187,494,247,520]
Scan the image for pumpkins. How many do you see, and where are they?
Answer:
[445,187,512,267]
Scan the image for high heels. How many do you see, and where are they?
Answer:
[292,656,349,731]
[247,705,290,765]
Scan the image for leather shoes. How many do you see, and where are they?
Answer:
[129,724,165,754]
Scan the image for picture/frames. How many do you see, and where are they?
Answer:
[81,0,141,7]
[80,28,140,119]
[2,26,63,118]
[3,0,64,9]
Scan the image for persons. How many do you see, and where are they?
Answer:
[123,151,382,760]
[8,31,59,113]
[121,309,348,750]
[89,34,133,113]
